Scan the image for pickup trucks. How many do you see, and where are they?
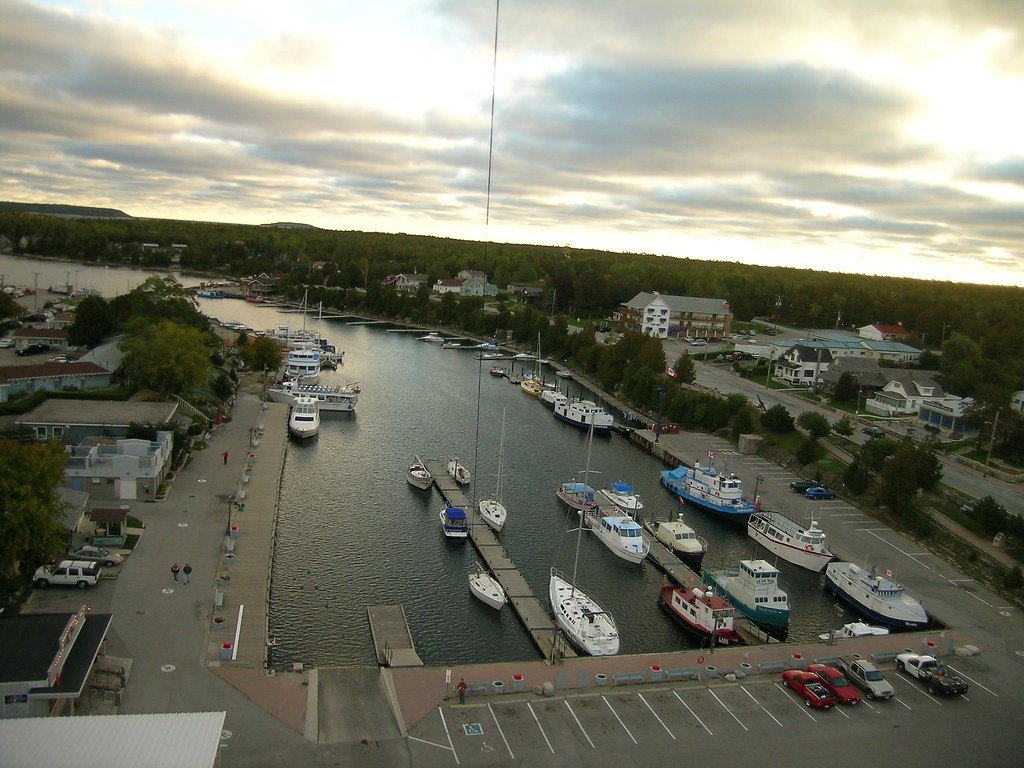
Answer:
[782,668,836,709]
[896,652,968,697]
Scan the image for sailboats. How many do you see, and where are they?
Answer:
[548,411,620,657]
[554,410,602,511]
[478,405,509,531]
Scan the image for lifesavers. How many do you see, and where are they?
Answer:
[806,544,814,552]
[714,491,720,498]
[336,385,340,389]
[613,506,619,513]
[603,511,609,516]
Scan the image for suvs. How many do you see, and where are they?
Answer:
[32,559,102,590]
[837,653,896,701]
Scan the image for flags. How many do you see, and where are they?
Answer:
[886,569,892,577]
[666,366,677,377]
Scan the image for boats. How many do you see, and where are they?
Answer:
[196,280,225,299]
[700,542,792,637]
[439,499,469,538]
[469,562,507,611]
[657,583,739,648]
[578,501,651,565]
[447,456,472,484]
[642,496,708,564]
[416,319,615,432]
[267,377,362,412]
[659,448,758,521]
[825,560,928,631]
[405,453,434,489]
[219,289,345,385]
[599,478,646,516]
[289,396,321,439]
[818,618,889,641]
[747,502,834,572]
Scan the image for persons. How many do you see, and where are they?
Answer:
[171,563,180,583]
[183,563,192,584]
[458,679,467,704]
[222,451,228,465]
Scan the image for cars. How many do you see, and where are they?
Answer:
[790,478,836,500]
[66,545,123,568]
[808,664,861,705]
[861,426,886,438]
[0,284,85,364]
[683,329,762,363]
